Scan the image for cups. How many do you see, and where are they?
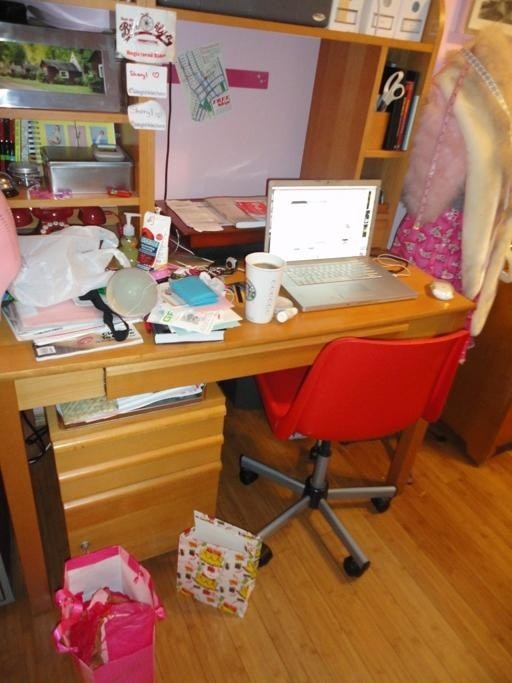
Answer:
[245,252,287,325]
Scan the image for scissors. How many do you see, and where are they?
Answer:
[377,71,405,112]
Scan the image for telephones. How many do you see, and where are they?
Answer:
[10,207,107,235]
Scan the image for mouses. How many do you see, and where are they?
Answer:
[430,279,454,301]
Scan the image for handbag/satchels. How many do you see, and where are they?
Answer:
[176,510,263,619]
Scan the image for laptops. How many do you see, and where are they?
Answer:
[263,178,417,314]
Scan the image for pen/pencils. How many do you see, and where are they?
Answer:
[144,314,153,335]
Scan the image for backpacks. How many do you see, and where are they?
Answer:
[51,545,166,683]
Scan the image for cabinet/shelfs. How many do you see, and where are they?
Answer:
[0,0,162,241]
[299,36,433,252]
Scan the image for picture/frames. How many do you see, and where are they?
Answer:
[0,22,122,112]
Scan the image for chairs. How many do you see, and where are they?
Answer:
[229,326,473,579]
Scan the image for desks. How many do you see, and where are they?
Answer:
[0,253,474,614]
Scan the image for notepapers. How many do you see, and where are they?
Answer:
[169,275,218,307]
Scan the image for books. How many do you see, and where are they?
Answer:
[151,275,243,344]
[0,291,144,362]
[205,195,268,229]
